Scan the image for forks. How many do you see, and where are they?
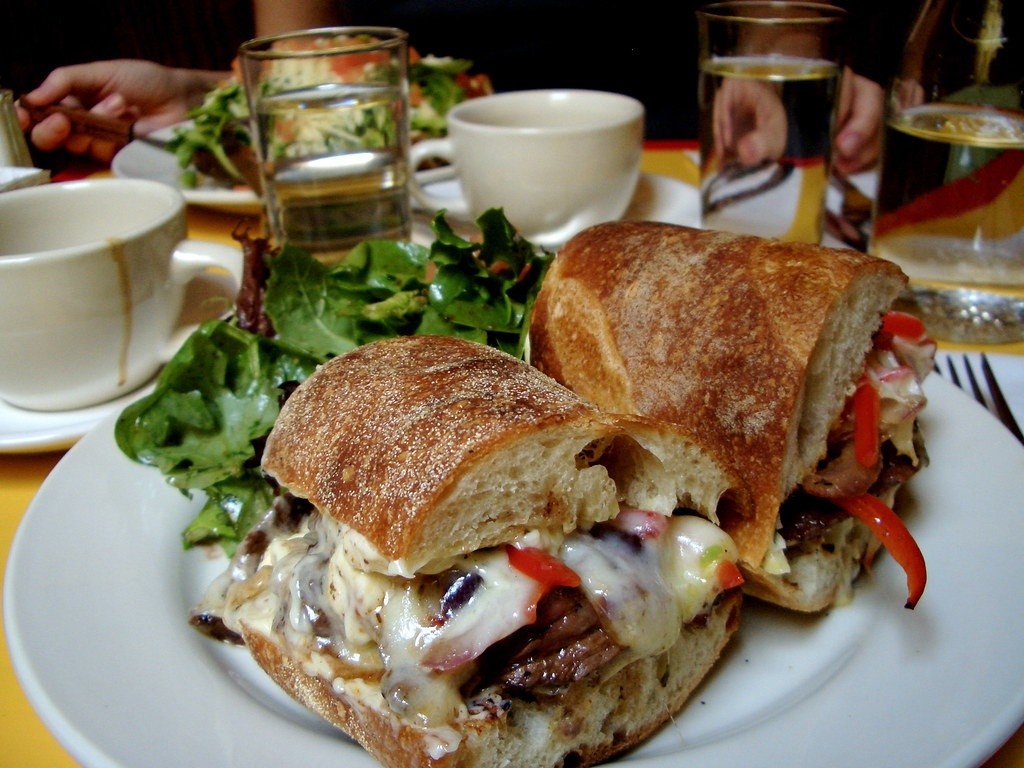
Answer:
[933,351,1024,447]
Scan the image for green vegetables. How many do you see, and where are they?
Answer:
[113,206,552,556]
[167,43,471,190]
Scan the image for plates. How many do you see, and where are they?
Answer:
[389,171,705,250]
[4,362,1024,768]
[111,118,459,214]
[0,273,245,454]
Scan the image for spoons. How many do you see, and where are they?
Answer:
[832,164,873,252]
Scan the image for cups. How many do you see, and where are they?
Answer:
[867,0,1024,345]
[0,166,51,193]
[0,90,34,167]
[0,181,244,412]
[698,2,844,244]
[409,89,645,245]
[240,27,412,265]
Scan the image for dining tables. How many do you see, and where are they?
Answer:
[0,143,1024,768]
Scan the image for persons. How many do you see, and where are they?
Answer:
[0,0,1024,176]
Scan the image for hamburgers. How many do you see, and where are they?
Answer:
[529,221,938,614]
[235,334,746,768]
